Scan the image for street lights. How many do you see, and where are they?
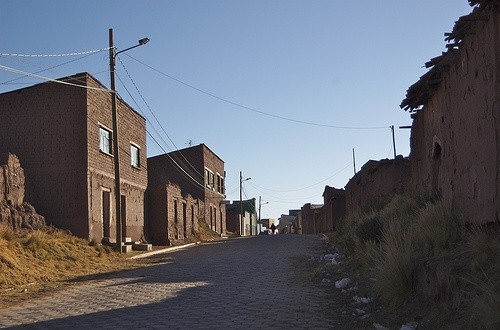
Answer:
[240,172,251,235]
[109,28,150,254]
[259,196,269,233]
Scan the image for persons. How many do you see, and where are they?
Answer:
[269,222,276,235]
